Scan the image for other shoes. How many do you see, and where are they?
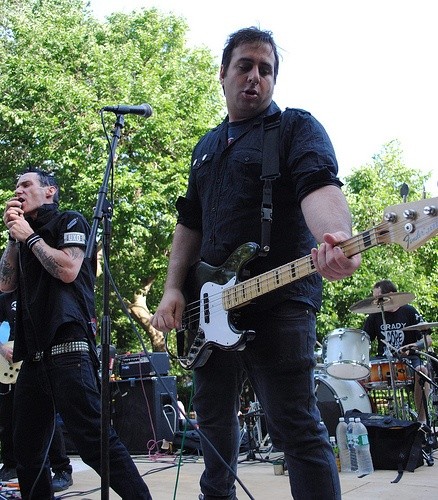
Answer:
[0,465,18,483]
[52,471,73,491]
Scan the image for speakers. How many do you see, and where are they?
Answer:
[59,421,80,455]
[112,375,178,455]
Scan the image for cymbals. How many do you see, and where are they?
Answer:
[396,320,438,332]
[349,290,416,315]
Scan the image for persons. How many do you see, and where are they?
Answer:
[151,25,361,500]
[0,170,151,500]
[358,280,433,423]
[176,393,185,431]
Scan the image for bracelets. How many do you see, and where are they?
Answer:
[25,233,42,250]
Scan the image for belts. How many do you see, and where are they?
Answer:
[31,342,90,362]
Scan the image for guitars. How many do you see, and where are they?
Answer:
[177,182,438,371]
[1,339,22,385]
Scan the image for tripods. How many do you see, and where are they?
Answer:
[237,383,275,464]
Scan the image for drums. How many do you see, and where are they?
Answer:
[366,357,415,389]
[313,373,375,444]
[321,327,373,382]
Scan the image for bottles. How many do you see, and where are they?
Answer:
[336,417,351,473]
[352,417,374,476]
[329,436,341,473]
[346,417,360,474]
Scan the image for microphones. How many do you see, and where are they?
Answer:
[398,345,413,354]
[248,378,255,401]
[103,103,153,118]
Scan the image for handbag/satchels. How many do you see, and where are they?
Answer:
[344,409,423,483]
